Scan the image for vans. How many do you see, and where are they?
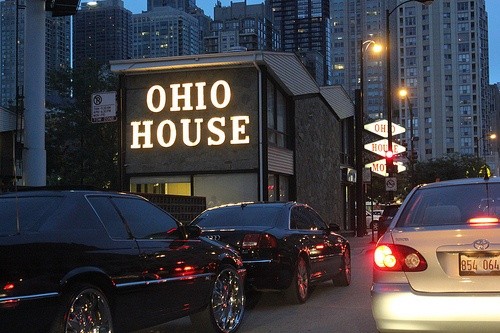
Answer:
[371,209,383,215]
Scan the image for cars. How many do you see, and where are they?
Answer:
[0,184,247,333]
[354,207,382,231]
[183,202,352,304]
[377,203,402,242]
[369,176,499,333]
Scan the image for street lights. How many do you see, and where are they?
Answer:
[69,0,98,97]
[399,89,413,152]
[358,39,383,238]
[385,0,434,201]
[477,133,497,162]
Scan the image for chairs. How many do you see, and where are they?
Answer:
[422,205,463,225]
